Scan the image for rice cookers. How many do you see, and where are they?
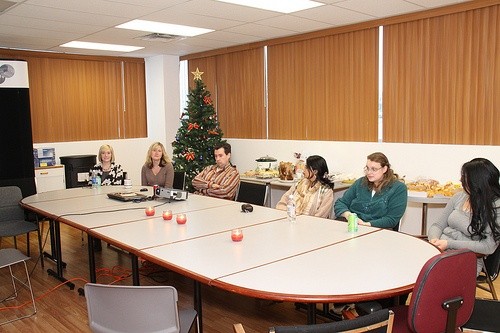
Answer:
[256,158,278,172]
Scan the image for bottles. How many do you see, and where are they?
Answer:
[92,172,98,189]
[287,194,296,221]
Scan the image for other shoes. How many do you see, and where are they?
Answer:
[330,304,358,320]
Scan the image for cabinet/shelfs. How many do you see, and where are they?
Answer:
[59,155,98,188]
[35,166,66,193]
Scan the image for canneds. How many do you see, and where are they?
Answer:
[153,185,160,196]
[348,213,358,233]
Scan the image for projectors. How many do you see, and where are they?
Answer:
[155,188,188,200]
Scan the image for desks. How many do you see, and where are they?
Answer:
[237,170,456,238]
[19,186,444,333]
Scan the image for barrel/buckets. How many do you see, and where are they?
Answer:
[59,154,97,189]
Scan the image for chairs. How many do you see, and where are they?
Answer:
[85,283,200,333]
[173,172,186,190]
[0,186,40,325]
[232,181,500,333]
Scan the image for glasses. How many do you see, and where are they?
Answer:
[364,166,383,171]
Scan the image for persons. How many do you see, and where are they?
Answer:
[275,155,335,218]
[342,156,500,322]
[140,141,175,190]
[191,140,240,200]
[87,143,124,186]
[317,152,408,318]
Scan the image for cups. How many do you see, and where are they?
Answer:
[145,206,155,216]
[124,179,133,189]
[231,229,243,242]
[176,213,186,224]
[163,210,172,220]
